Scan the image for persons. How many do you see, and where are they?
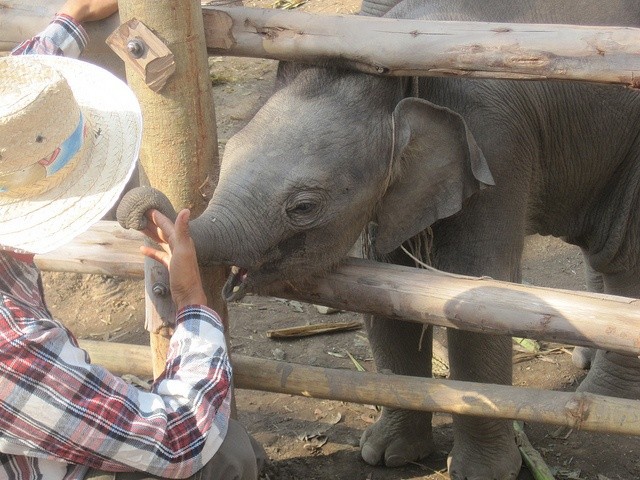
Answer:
[1,0,257,480]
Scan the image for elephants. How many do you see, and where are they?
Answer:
[117,0,639,480]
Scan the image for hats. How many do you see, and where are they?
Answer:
[0,53,145,256]
